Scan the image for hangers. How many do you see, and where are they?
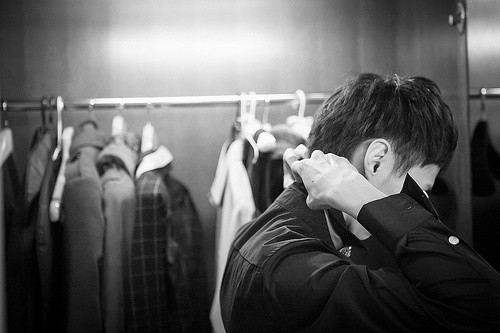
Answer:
[481,88,487,122]
[0,89,307,223]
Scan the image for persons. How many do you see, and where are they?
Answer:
[219,73,500,333]
